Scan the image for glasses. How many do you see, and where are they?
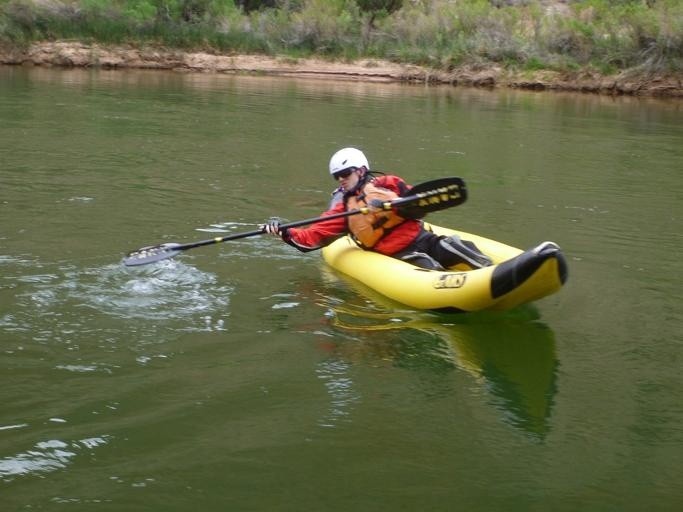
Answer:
[333,169,353,180]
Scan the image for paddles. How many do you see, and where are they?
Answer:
[125,177,467,267]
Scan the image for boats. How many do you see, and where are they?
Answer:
[323,218,570,315]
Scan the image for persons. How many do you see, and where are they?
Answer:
[258,146,493,272]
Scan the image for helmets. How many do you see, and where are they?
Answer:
[329,147,370,175]
